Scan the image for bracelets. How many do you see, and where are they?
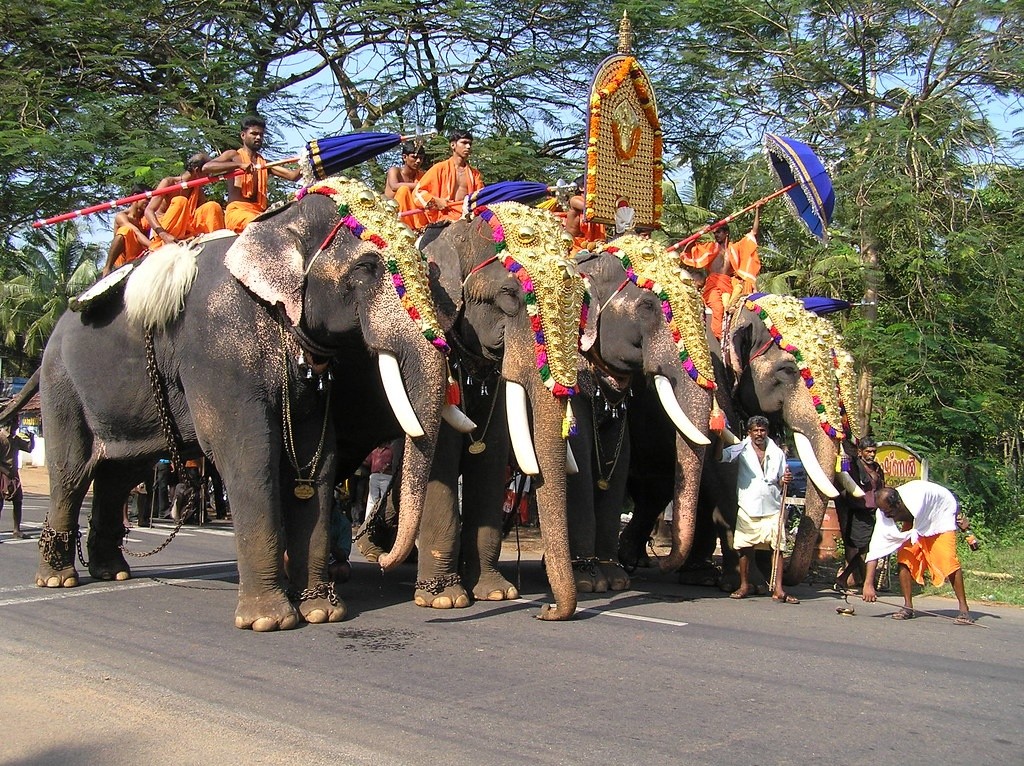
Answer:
[154,227,165,235]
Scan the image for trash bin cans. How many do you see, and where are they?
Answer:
[812,501,842,564]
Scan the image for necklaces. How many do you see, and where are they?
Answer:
[180,175,183,183]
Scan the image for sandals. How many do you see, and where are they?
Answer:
[892,607,915,620]
[954,612,974,625]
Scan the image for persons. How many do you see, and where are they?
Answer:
[863,479,974,624]
[333,438,394,526]
[682,199,761,340]
[567,174,607,246]
[834,437,884,591]
[144,153,224,249]
[384,140,424,230]
[505,464,540,526]
[103,183,153,276]
[202,116,302,232]
[411,128,485,223]
[0,406,34,538]
[119,453,229,527]
[712,415,800,605]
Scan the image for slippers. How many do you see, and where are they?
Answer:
[729,589,754,598]
[771,593,800,604]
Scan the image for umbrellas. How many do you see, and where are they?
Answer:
[399,181,565,218]
[802,297,875,316]
[31,125,430,227]
[666,131,835,252]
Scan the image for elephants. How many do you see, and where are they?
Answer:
[36,176,450,630]
[540,237,719,591]
[680,292,855,593]
[416,200,585,621]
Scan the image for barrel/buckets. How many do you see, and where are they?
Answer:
[813,505,841,558]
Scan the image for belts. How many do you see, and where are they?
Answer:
[372,471,393,475]
